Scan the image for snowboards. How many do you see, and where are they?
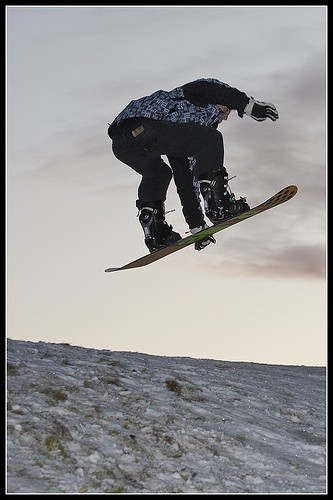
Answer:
[105,184,298,273]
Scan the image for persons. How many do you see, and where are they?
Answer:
[107,78,279,253]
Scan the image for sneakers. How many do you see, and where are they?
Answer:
[199,166,249,225]
[136,200,182,252]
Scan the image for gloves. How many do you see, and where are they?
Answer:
[245,96,279,122]
[190,224,216,251]
